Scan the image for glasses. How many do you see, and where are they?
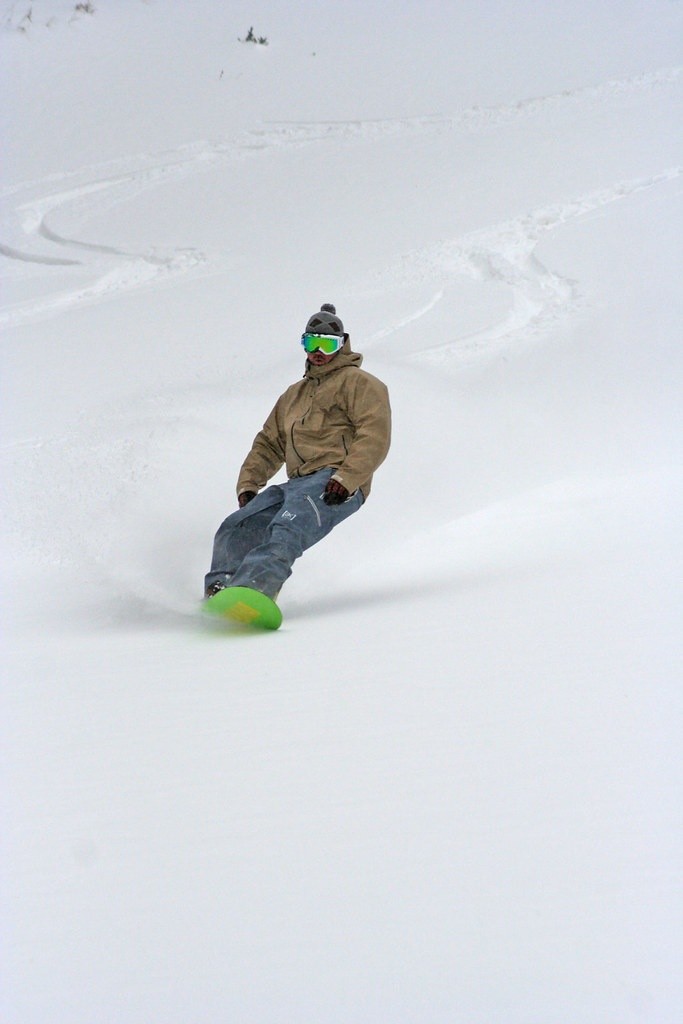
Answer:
[302,333,344,356]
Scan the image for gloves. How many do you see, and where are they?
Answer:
[238,491,256,509]
[324,479,349,505]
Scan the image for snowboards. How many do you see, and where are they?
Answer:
[205,585,286,629]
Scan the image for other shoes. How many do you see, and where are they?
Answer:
[207,580,226,598]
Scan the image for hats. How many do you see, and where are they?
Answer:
[306,303,344,337]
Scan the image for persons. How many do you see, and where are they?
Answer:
[207,300,393,605]
